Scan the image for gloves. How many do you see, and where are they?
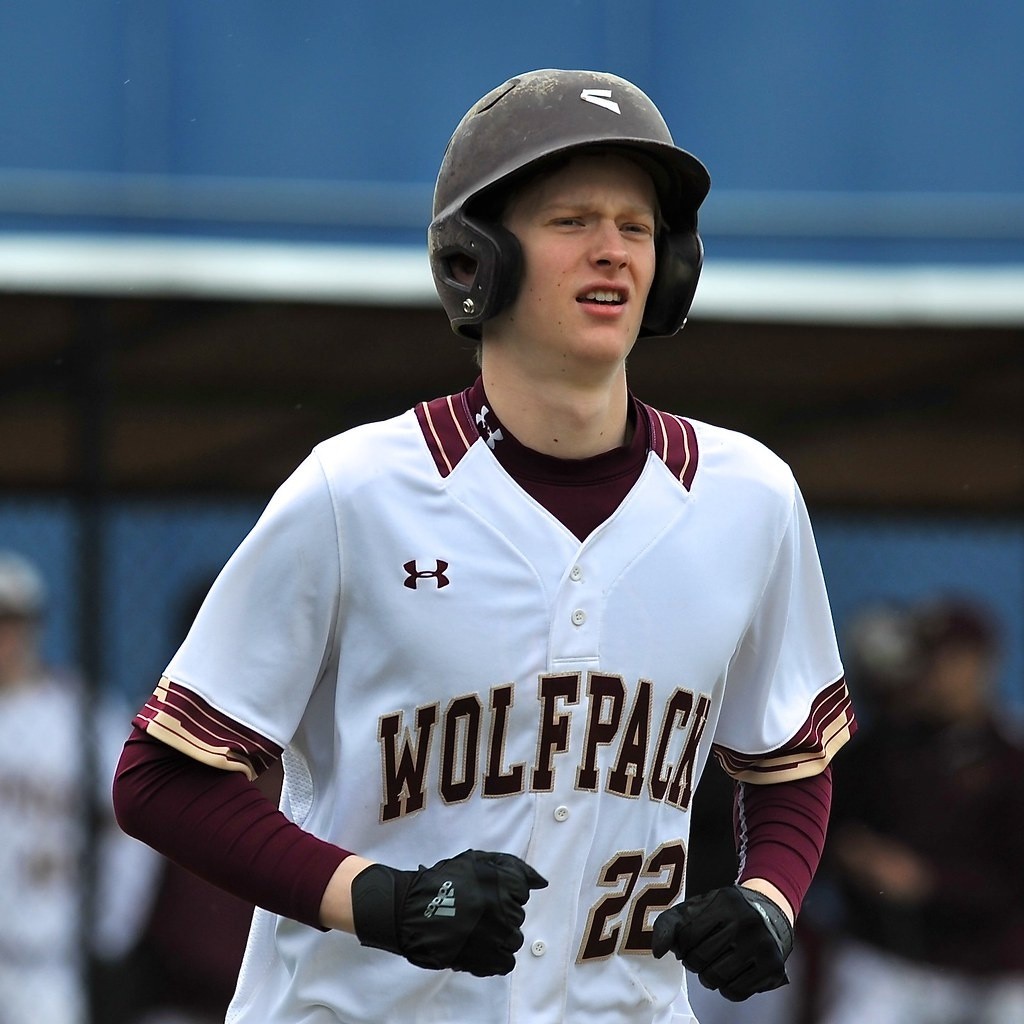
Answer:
[651,884,795,999]
[350,851,549,979]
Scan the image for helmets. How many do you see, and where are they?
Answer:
[431,68,712,343]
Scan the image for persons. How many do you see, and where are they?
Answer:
[0,546,165,1024]
[815,584,1024,1024]
[114,67,858,1024]
[149,568,283,1021]
